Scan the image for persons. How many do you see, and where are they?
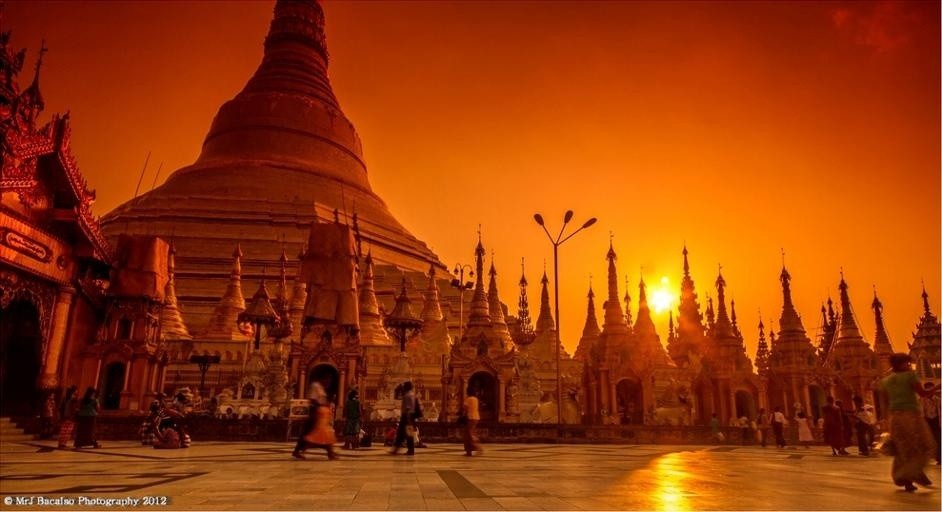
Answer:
[37,390,56,437]
[139,372,487,461]
[57,383,80,451]
[918,382,940,465]
[708,393,877,457]
[878,353,933,493]
[74,386,102,448]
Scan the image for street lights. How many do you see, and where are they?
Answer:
[198,356,212,396]
[533,209,598,423]
[450,263,477,336]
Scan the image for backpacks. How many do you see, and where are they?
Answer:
[414,397,425,418]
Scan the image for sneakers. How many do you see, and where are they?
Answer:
[460,451,472,457]
[915,478,932,486]
[830,448,871,459]
[904,484,918,492]
[290,440,428,463]
